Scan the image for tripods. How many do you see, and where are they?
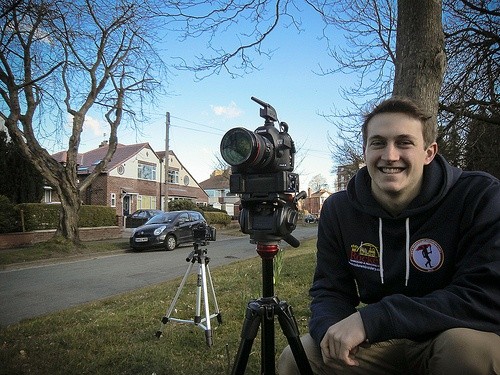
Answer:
[230,193,314,375]
[155,242,222,348]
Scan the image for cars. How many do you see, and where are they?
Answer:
[129,211,208,251]
[125,209,165,227]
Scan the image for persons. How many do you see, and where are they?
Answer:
[277,94,500,375]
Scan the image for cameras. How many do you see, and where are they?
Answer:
[220,96,300,197]
[191,222,217,244]
